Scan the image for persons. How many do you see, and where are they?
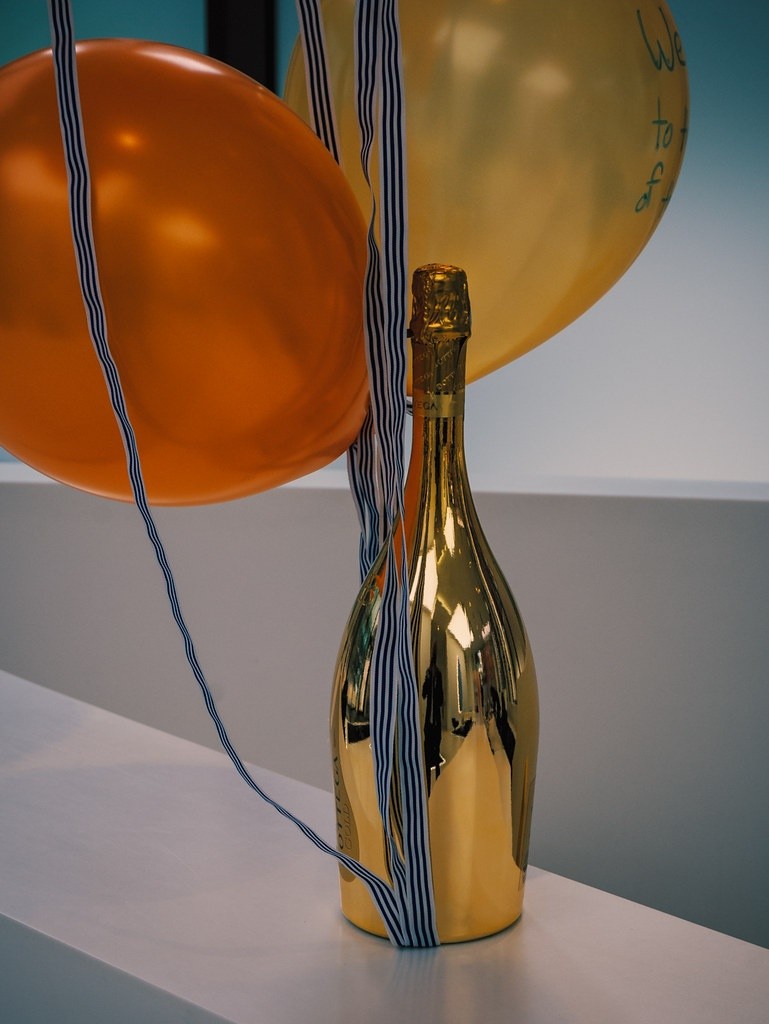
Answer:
[422,642,444,797]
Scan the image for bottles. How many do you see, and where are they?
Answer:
[332,266,539,943]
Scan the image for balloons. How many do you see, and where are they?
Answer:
[284,0,691,397]
[0,38,371,507]
